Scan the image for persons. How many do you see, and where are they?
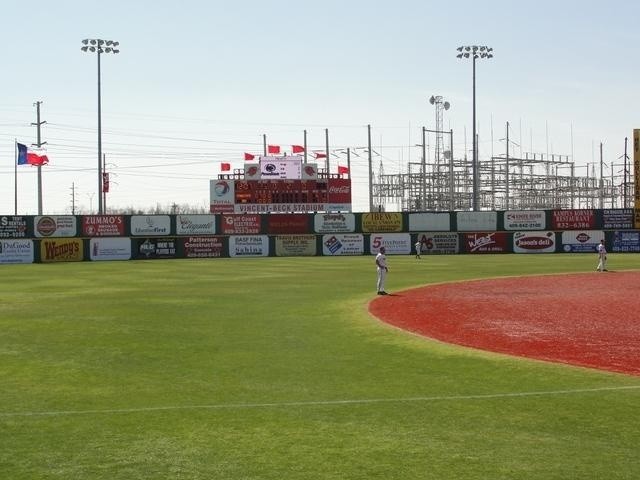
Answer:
[415,241,422,259]
[596,239,608,272]
[376,246,390,295]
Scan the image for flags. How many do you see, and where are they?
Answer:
[244,152,255,160]
[221,163,230,171]
[338,167,349,174]
[292,145,304,153]
[17,142,49,166]
[269,146,280,153]
[315,153,326,158]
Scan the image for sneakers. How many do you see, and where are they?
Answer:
[378,291,388,295]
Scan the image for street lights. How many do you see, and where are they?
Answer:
[457,44,492,212]
[80,38,119,215]
